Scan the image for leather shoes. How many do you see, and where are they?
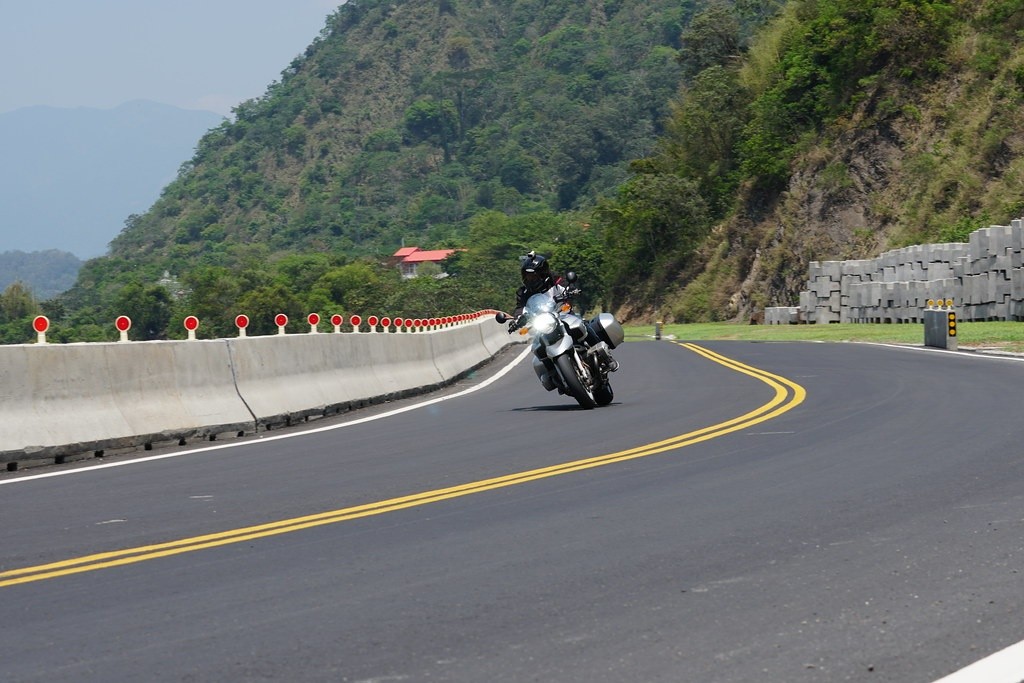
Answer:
[607,356,620,373]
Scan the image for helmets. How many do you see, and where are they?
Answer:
[520,251,552,293]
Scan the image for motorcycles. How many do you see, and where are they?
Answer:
[496,271,625,409]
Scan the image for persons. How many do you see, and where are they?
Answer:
[509,250,619,395]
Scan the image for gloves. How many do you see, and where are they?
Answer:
[508,320,519,334]
[563,285,579,298]
